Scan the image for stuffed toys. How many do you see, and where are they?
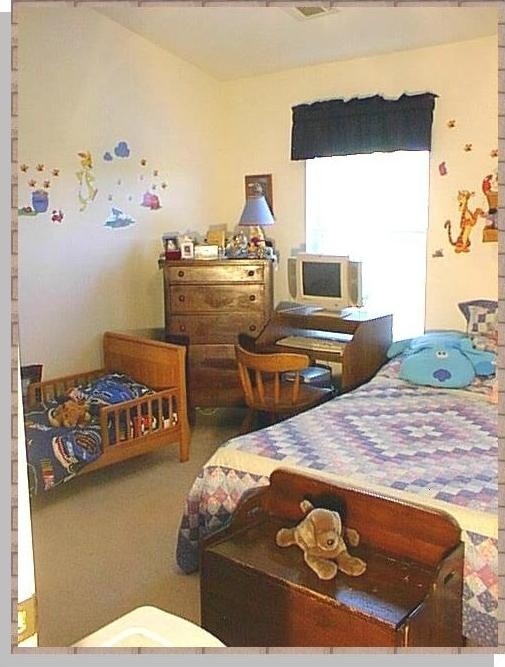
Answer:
[48,398,91,426]
[384,329,497,391]
[275,498,370,580]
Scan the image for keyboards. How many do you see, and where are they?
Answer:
[275,335,347,355]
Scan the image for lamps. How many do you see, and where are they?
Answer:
[236,195,275,258]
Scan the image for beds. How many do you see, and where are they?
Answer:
[215,342,498,645]
[24,331,190,494]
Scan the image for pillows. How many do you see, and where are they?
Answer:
[53,368,167,429]
[377,299,499,399]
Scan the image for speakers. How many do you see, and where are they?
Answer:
[288,258,296,301]
[348,262,363,307]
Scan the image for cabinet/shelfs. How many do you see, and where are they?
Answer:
[198,468,464,648]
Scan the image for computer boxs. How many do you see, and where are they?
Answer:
[281,367,330,386]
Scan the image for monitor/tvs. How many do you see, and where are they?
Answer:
[296,254,351,318]
